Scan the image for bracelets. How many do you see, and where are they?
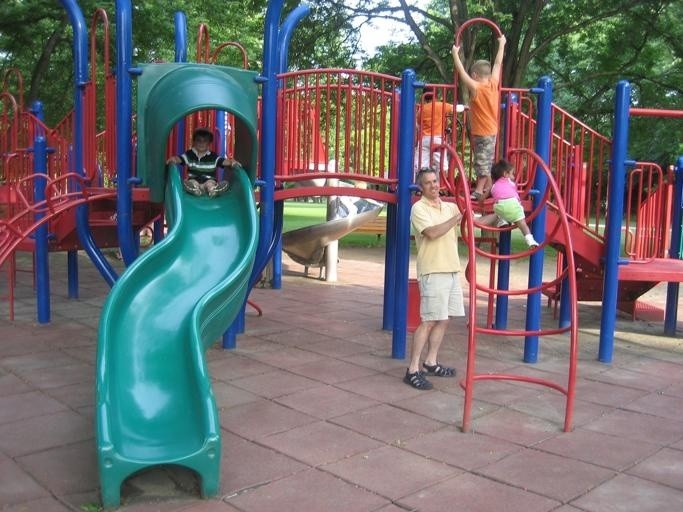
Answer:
[455,214,461,221]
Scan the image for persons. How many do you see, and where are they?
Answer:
[402,167,501,391]
[451,33,507,201]
[164,126,245,200]
[413,86,471,184]
[478,159,541,251]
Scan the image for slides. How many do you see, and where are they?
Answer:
[281,179,383,268]
[95,163,259,510]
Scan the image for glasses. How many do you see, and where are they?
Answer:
[418,167,435,176]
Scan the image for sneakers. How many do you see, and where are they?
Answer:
[209,181,229,199]
[183,181,202,197]
[528,241,540,249]
[496,219,509,228]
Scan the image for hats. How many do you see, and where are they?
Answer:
[193,128,214,143]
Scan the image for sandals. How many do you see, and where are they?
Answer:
[403,362,456,390]
[471,193,481,200]
